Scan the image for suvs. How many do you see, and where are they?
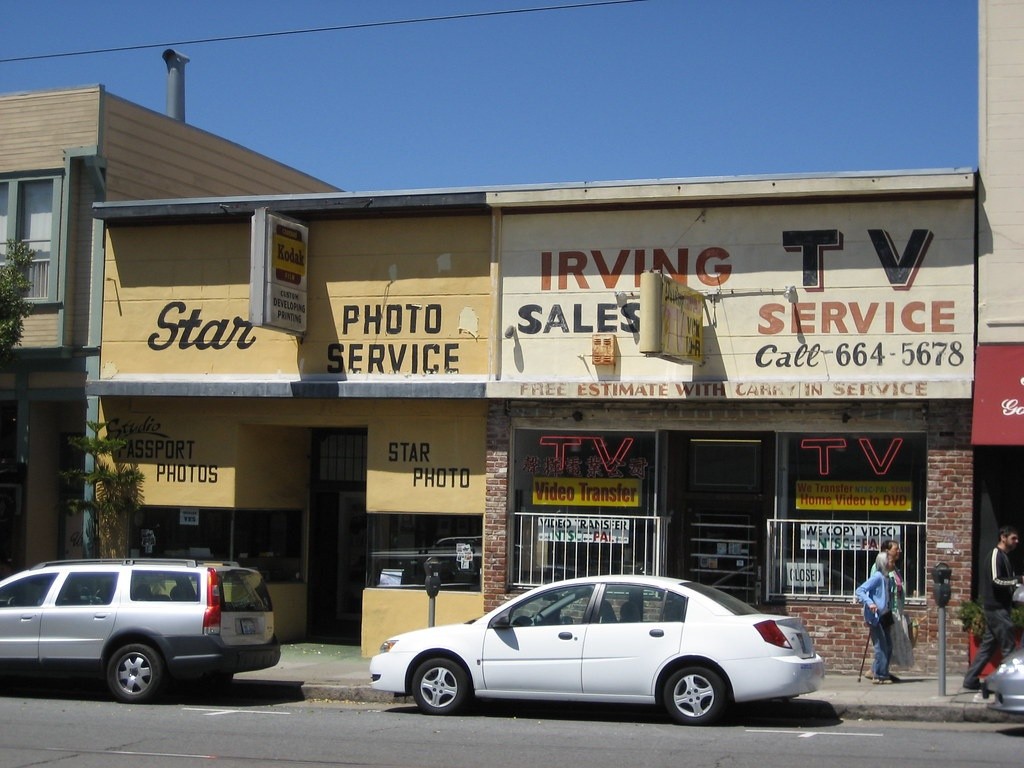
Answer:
[1,558,282,703]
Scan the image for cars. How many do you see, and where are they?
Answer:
[419,535,589,588]
[985,646,1024,714]
[374,574,826,727]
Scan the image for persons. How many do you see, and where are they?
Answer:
[854,540,907,684]
[962,524,1023,690]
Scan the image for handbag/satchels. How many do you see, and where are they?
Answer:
[1011,583,1024,603]
[879,610,895,630]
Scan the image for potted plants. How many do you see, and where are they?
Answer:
[960,599,1024,678]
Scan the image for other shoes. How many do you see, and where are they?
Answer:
[889,675,900,683]
[963,678,982,690]
[865,668,875,679]
[873,677,892,684]
